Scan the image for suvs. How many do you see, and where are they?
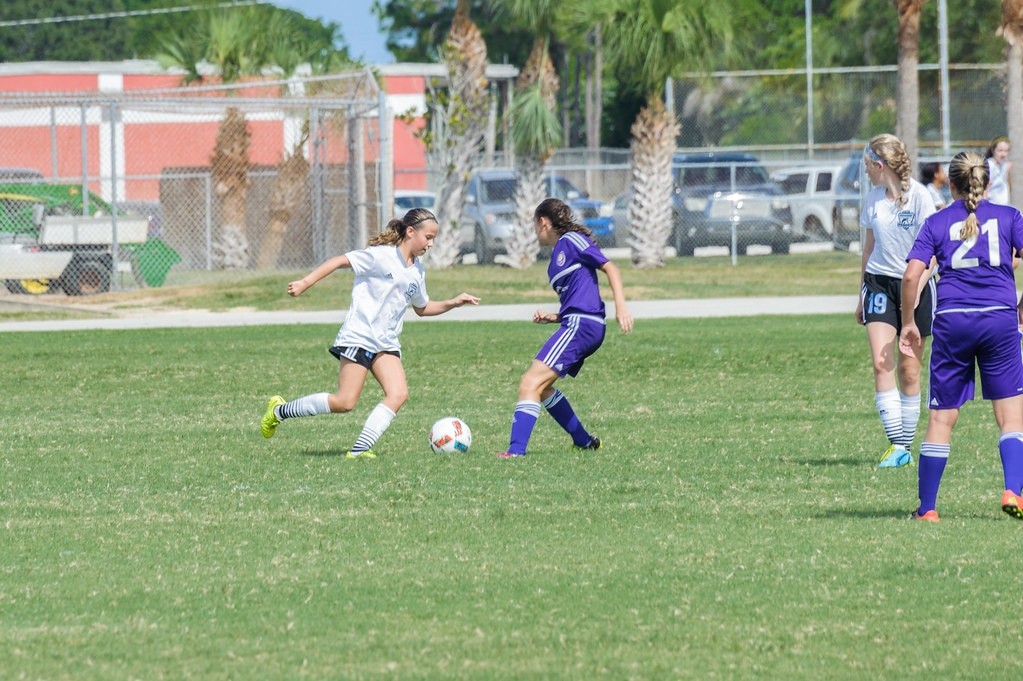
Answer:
[612,150,797,258]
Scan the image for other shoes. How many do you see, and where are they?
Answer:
[495,452,517,459]
[571,435,603,452]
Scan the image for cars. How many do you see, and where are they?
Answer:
[762,164,846,246]
[454,163,583,268]
[544,172,617,250]
[831,150,952,252]
[391,189,438,223]
[106,198,165,241]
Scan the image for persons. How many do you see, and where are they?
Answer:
[855,130,1023,520]
[261,208,481,458]
[498,198,634,459]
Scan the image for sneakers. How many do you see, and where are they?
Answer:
[260,396,286,439]
[1002,490,1023,519]
[878,445,916,470]
[910,508,940,522]
[346,450,378,460]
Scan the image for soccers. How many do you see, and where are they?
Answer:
[429,416,473,454]
